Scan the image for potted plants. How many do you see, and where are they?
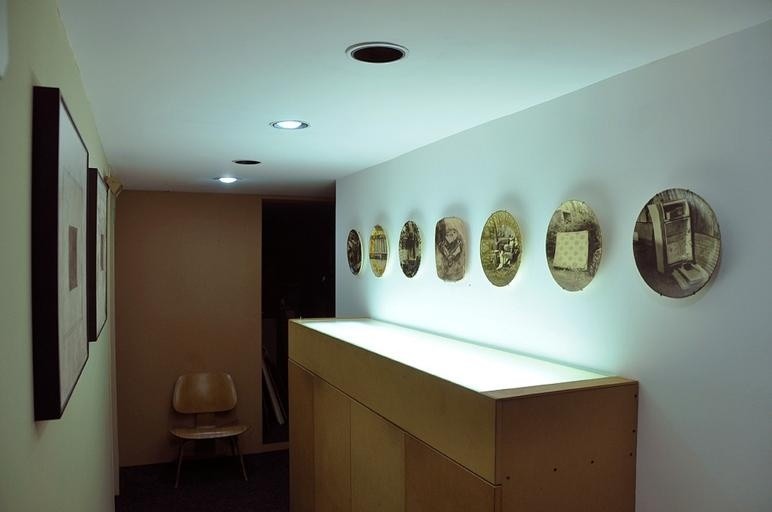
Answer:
[33,84,109,423]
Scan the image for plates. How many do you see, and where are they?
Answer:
[287,357,638,512]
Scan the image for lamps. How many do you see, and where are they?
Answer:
[170,371,250,489]
